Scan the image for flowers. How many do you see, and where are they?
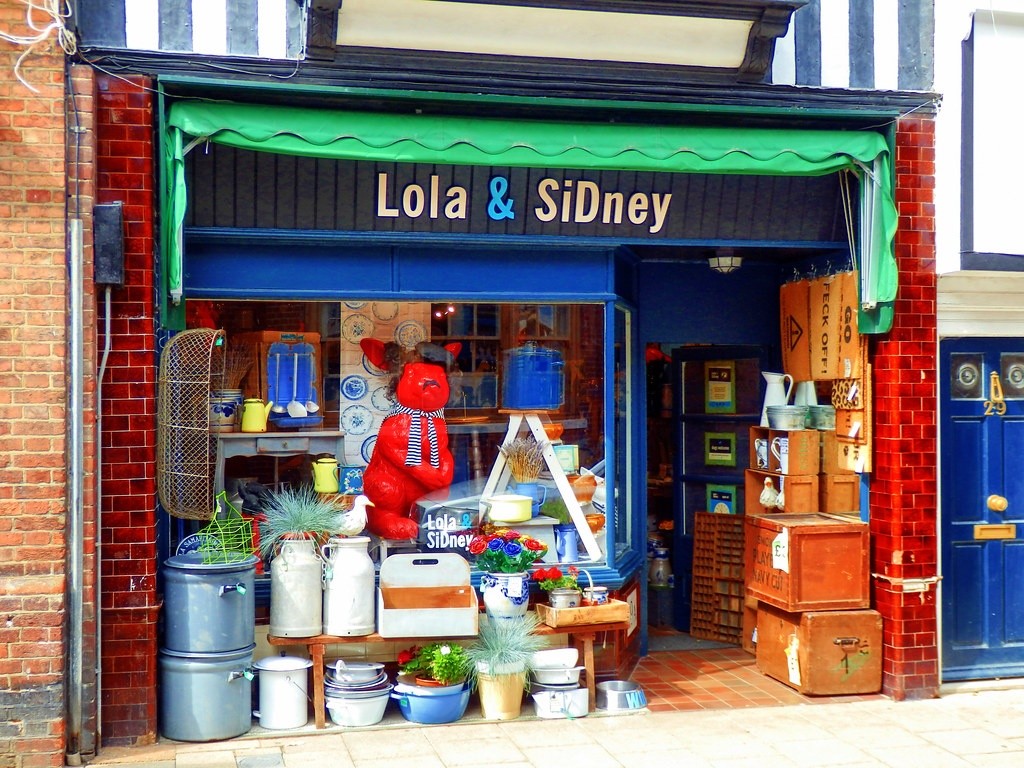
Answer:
[468,528,548,572]
[396,641,469,683]
[532,566,581,590]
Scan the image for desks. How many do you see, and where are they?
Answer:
[213,418,588,564]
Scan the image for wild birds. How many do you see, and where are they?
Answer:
[759,477,780,514]
[776,478,785,513]
[333,495,375,539]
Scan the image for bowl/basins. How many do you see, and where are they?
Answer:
[391,671,474,724]
[527,646,647,710]
[324,660,394,727]
[766,405,836,430]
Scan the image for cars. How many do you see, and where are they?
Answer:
[408,433,605,555]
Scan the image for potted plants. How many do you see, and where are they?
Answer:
[461,609,550,720]
[256,482,346,562]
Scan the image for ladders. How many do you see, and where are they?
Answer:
[479,410,605,562]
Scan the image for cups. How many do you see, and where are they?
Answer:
[771,437,789,475]
[755,439,767,469]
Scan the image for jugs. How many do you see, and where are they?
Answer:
[334,464,367,495]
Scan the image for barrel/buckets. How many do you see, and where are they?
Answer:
[269,540,322,638]
[158,553,263,741]
[321,536,375,637]
[253,652,313,730]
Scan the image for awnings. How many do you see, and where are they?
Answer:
[164,101,898,336]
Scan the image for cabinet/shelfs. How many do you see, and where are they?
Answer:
[742,424,821,656]
[822,431,860,515]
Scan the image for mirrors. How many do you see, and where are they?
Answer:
[786,376,869,524]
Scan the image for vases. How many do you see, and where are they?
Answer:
[549,588,581,609]
[416,673,450,687]
[480,571,530,627]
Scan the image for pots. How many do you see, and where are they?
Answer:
[480,494,533,522]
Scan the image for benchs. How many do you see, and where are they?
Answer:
[267,621,629,728]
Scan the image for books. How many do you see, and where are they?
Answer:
[704,359,737,414]
[706,484,737,515]
[704,432,736,467]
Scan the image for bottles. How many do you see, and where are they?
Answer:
[775,476,785,511]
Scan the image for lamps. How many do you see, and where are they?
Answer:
[707,248,743,272]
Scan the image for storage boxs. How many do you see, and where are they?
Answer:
[756,599,882,695]
[536,598,630,628]
[742,513,869,613]
[376,553,478,638]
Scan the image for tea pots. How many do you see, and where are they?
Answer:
[555,528,579,564]
[760,370,793,427]
[312,458,339,495]
[237,398,274,432]
[508,483,547,517]
